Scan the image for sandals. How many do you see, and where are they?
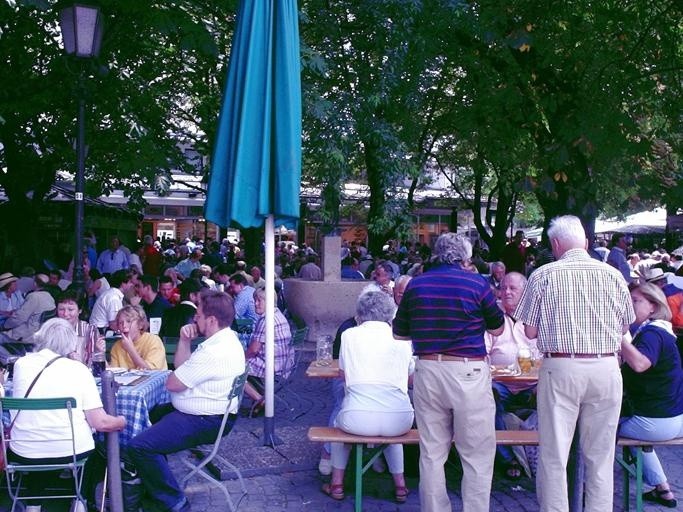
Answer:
[322,483,344,499]
[248,399,265,418]
[505,458,522,481]
[642,488,676,507]
[395,487,410,502]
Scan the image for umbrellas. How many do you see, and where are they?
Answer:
[201,2,303,448]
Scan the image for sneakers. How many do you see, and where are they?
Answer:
[319,459,331,475]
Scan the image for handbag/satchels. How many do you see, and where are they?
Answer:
[0,433,10,470]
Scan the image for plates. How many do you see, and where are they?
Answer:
[103,367,127,375]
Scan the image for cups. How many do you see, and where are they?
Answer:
[91,353,106,376]
[515,348,531,374]
[315,333,334,367]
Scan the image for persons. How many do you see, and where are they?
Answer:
[71,231,323,290]
[512,213,638,511]
[1,268,295,512]
[319,233,683,512]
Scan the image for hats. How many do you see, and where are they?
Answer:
[0,272,19,288]
[645,268,669,282]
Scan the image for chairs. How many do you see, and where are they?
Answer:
[162,336,205,365]
[105,337,121,362]
[176,373,250,512]
[245,326,308,427]
[0,342,35,356]
[40,307,58,325]
[0,397,91,512]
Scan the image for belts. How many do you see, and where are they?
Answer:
[545,353,614,358]
[419,354,484,361]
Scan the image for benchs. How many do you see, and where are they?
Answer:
[308,425,683,512]
